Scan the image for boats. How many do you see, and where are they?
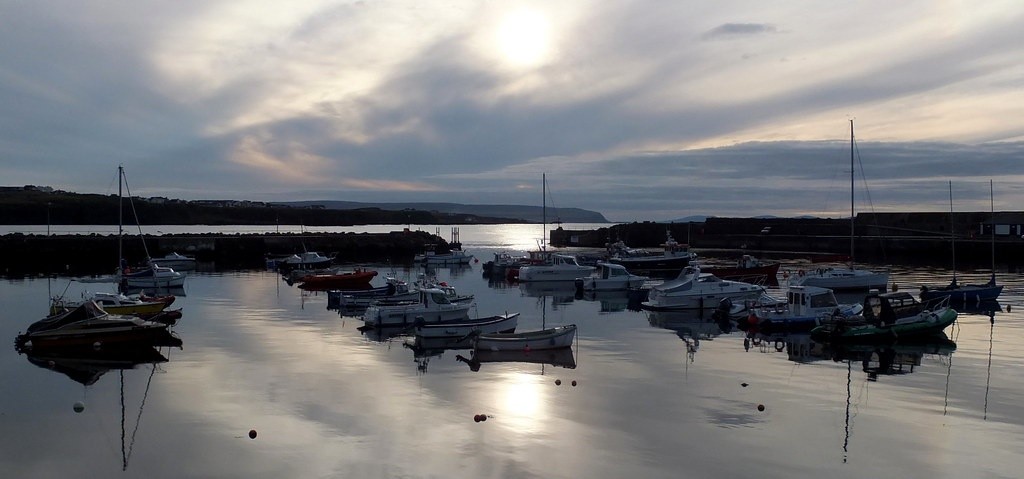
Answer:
[287,266,340,277]
[427,250,474,266]
[403,336,475,350]
[414,252,435,262]
[118,284,186,298]
[300,265,474,328]
[146,250,196,269]
[17,343,170,387]
[278,251,336,275]
[458,324,577,351]
[118,265,187,289]
[455,347,576,373]
[413,309,521,339]
[483,248,958,345]
[17,302,166,345]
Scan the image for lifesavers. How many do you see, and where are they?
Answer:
[775,339,785,350]
[798,270,805,277]
[783,269,790,277]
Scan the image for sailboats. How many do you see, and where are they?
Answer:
[503,172,572,281]
[114,332,185,473]
[785,119,895,297]
[919,180,1004,303]
[51,165,175,318]
